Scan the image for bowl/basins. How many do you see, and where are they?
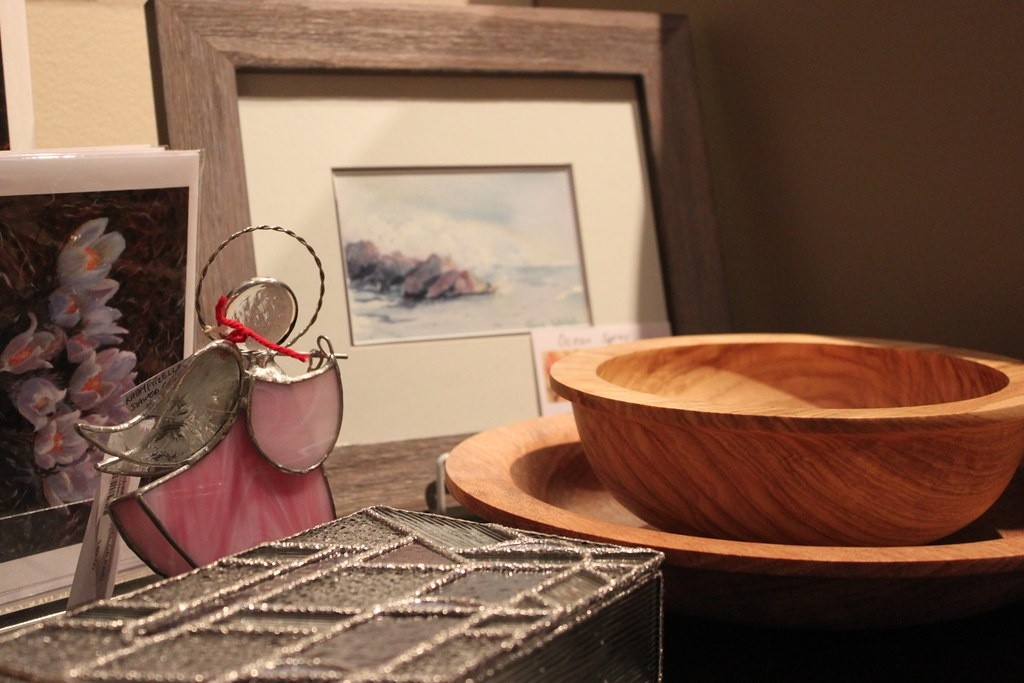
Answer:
[548,331,1023,545]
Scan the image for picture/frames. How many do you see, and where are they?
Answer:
[145,0,738,526]
